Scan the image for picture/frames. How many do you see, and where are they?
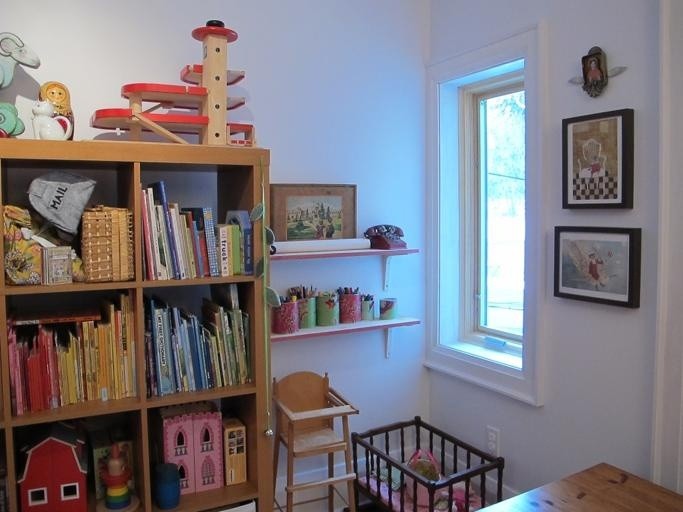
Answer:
[271,183,357,241]
[553,226,644,310]
[561,108,635,210]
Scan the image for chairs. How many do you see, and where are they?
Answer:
[272,371,357,512]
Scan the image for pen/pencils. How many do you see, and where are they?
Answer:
[362,294,375,301]
[338,286,361,294]
[315,287,337,296]
[290,287,312,298]
[279,295,296,302]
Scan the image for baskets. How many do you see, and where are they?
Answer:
[82,205,134,283]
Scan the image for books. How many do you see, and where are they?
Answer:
[139,180,239,281]
[7,283,250,418]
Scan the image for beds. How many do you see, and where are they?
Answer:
[351,415,504,512]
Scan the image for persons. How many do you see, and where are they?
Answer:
[587,61,602,81]
[326,218,335,238]
[316,220,326,240]
[588,252,605,289]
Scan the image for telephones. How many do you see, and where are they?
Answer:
[365,225,406,249]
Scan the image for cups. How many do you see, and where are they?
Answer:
[271,292,398,337]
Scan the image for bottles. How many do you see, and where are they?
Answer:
[154,462,180,511]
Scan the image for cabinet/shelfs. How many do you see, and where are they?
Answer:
[270,248,422,359]
[1,137,270,511]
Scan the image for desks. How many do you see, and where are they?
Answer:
[476,462,683,512]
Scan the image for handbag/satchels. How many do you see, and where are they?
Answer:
[2,206,85,285]
[27,171,97,246]
[405,450,441,506]
